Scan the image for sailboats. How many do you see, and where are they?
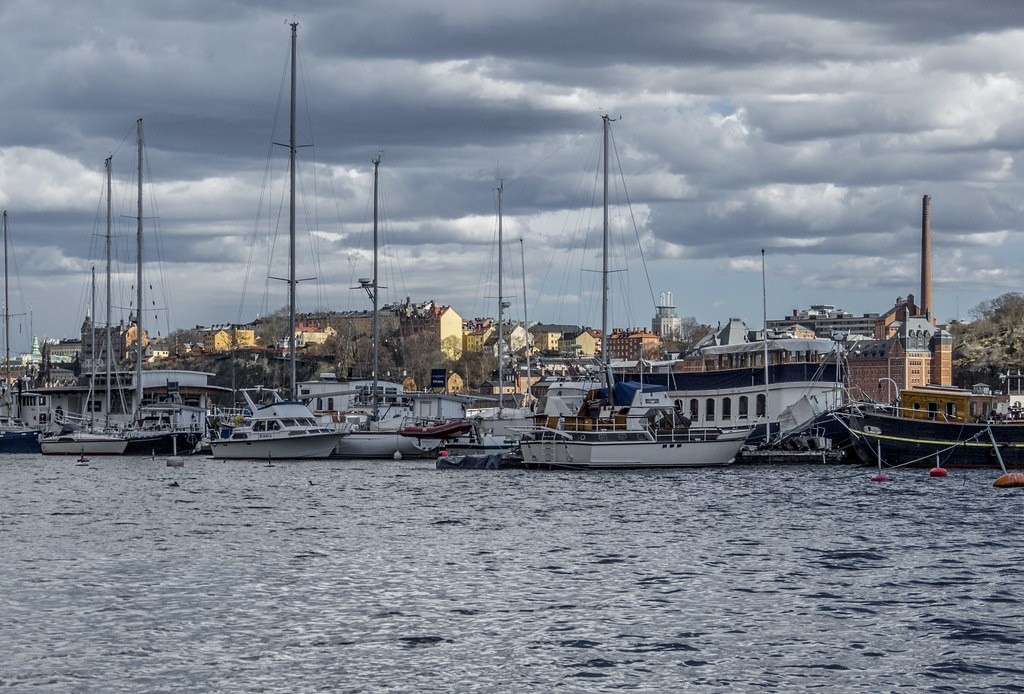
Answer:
[503,114,760,472]
[0,20,1024,489]
[203,23,356,462]
[34,157,131,455]
